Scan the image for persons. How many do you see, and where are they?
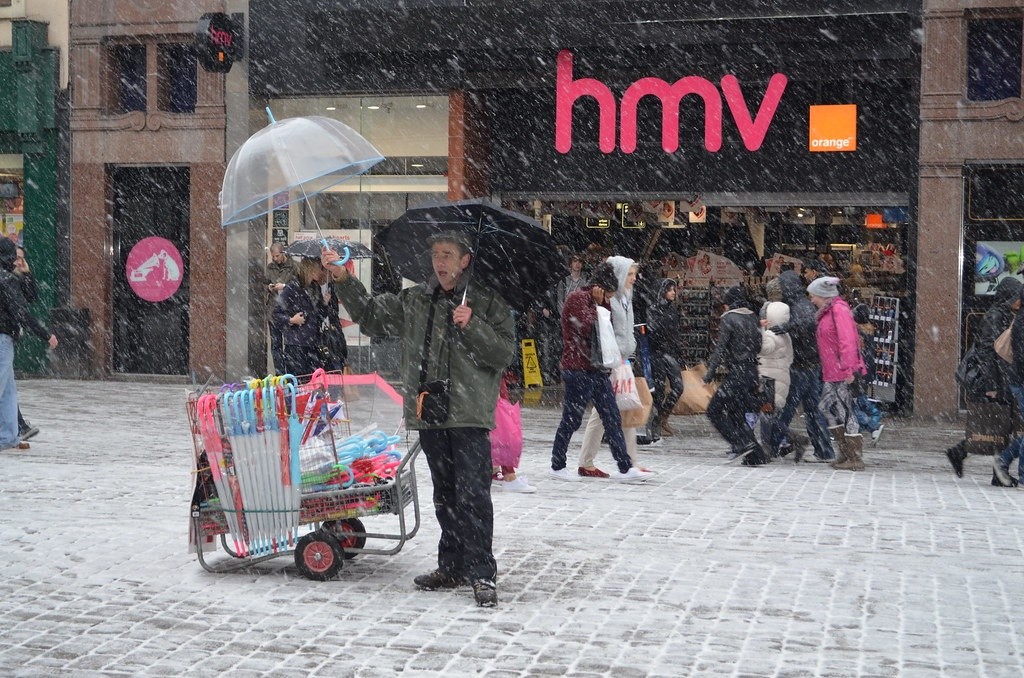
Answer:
[575,254,658,478]
[524,246,585,385]
[550,261,656,482]
[272,255,349,403]
[702,244,886,470]
[318,232,518,607]
[602,274,686,443]
[0,236,59,451]
[943,272,1024,487]
[265,242,303,376]
[488,376,538,493]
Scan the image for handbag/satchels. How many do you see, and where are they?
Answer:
[994,316,1016,363]
[317,316,343,364]
[953,314,994,395]
[416,381,451,424]
[591,306,622,376]
[663,361,718,414]
[602,360,654,428]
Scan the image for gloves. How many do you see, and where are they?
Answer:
[701,369,717,385]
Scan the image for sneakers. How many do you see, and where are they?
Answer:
[577,466,611,482]
[472,575,496,607]
[500,476,537,494]
[415,569,476,592]
[618,467,654,483]
[489,478,504,489]
[548,467,581,482]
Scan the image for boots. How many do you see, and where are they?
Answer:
[827,423,849,466]
[834,432,864,472]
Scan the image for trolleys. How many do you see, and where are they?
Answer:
[190,434,423,582]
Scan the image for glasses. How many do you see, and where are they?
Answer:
[570,256,585,264]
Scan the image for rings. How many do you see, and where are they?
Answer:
[325,296,327,298]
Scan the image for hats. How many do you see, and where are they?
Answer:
[426,230,473,253]
[805,274,841,298]
[852,304,869,324]
[724,285,744,306]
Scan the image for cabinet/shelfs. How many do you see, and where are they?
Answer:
[863,296,900,403]
[674,288,713,366]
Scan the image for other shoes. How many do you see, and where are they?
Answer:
[723,434,836,465]
[990,473,1020,488]
[944,445,964,477]
[871,424,883,447]
[16,422,39,440]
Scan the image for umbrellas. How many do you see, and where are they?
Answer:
[195,367,402,562]
[375,195,572,333]
[215,108,387,265]
[282,236,373,294]
